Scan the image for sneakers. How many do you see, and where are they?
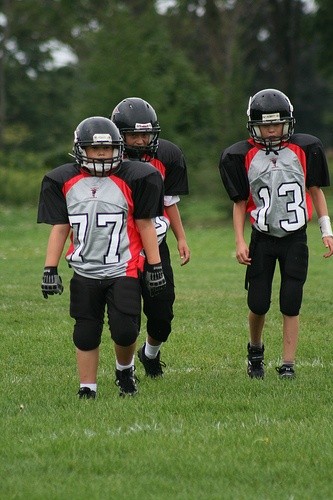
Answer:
[76,387,96,400]
[137,343,163,379]
[115,367,138,396]
[247,343,266,380]
[275,362,296,380]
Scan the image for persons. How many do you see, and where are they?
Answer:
[111,97,190,382]
[36,117,169,402]
[220,90,333,380]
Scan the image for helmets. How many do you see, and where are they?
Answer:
[247,88,295,151]
[72,116,124,175]
[110,96,162,162]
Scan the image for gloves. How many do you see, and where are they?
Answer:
[142,263,167,297]
[41,265,65,299]
